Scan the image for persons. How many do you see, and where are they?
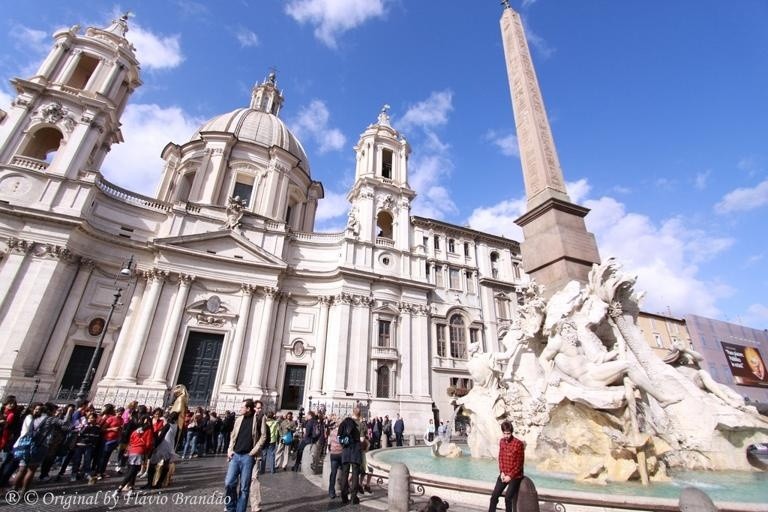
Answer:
[666,340,746,412]
[428,419,434,442]
[467,339,529,387]
[0,395,404,512]
[170,384,188,458]
[438,421,450,435]
[417,496,449,512]
[536,318,686,410]
[224,195,246,230]
[460,423,471,436]
[489,422,524,512]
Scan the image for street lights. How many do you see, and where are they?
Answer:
[75,254,137,409]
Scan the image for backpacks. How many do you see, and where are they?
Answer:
[12,417,48,461]
[257,414,271,447]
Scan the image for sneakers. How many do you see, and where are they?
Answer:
[181,450,224,459]
[275,466,299,472]
[327,485,372,505]
[112,490,122,499]
[119,484,134,492]
[39,474,110,486]
[136,471,145,476]
[140,472,148,479]
[5,486,27,494]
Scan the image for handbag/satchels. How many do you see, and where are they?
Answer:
[282,432,293,444]
